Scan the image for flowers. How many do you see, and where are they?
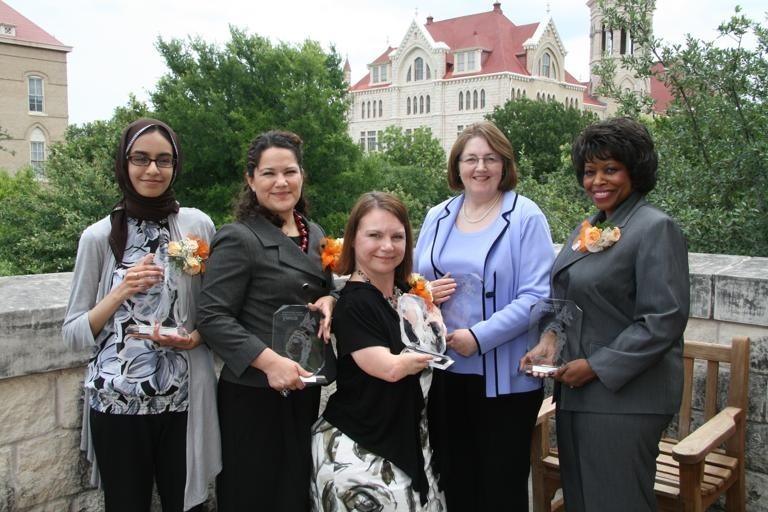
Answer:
[576,218,621,254]
[168,233,213,274]
[402,272,444,308]
[321,236,360,273]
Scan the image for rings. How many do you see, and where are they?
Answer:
[134,272,140,279]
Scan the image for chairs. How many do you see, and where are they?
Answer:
[526,336,750,512]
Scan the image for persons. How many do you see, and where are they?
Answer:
[57,115,219,508]
[515,114,693,511]
[404,119,560,512]
[193,127,343,512]
[303,190,457,512]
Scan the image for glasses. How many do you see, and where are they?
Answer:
[458,155,504,166]
[125,152,175,169]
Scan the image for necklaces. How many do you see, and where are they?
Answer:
[355,266,372,285]
[462,191,501,223]
[294,210,310,250]
